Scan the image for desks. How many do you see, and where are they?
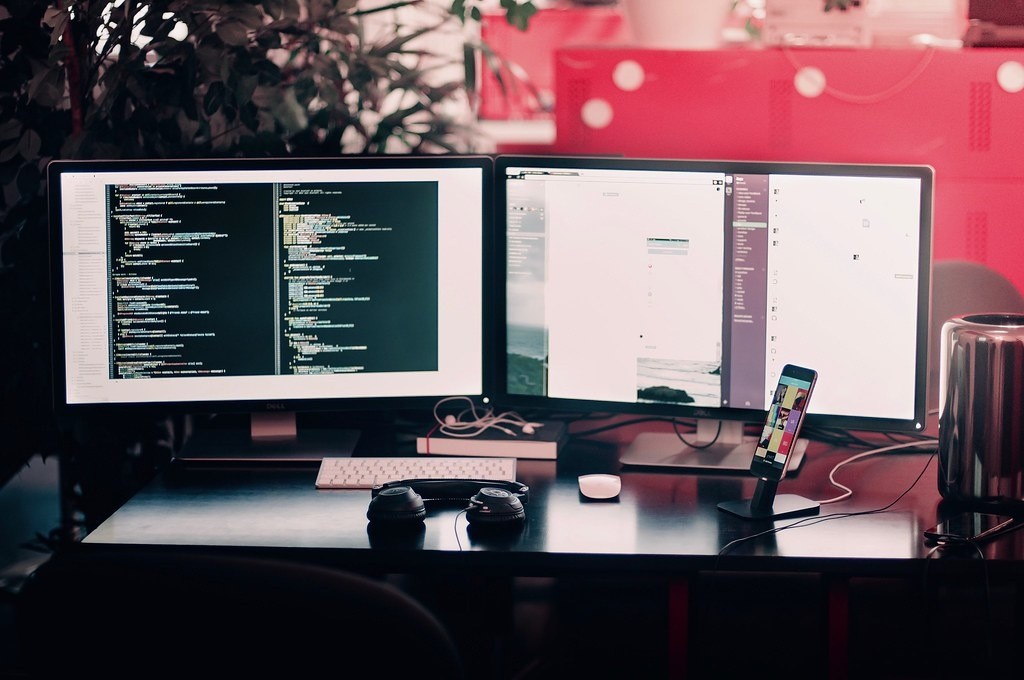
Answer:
[81,399,1023,680]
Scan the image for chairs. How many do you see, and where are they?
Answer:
[82,556,465,679]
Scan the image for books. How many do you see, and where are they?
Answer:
[416,417,570,458]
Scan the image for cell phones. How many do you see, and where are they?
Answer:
[751,364,817,481]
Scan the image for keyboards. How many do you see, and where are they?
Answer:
[315,456,517,488]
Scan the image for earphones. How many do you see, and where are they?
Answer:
[445,415,467,426]
[522,423,541,434]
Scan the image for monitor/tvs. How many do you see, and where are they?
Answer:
[46,154,495,463]
[495,152,936,471]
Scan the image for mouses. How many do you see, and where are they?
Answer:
[579,474,621,498]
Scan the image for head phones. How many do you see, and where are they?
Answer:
[366,478,529,528]
